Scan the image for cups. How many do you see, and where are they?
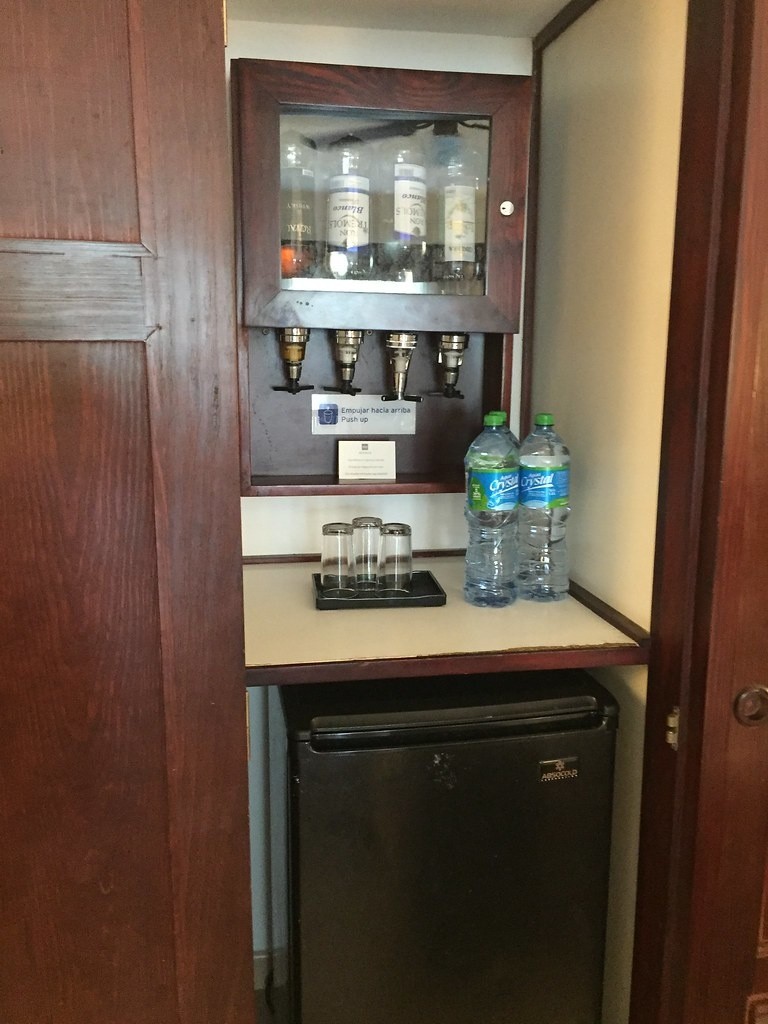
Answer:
[348,517,382,591]
[320,523,358,599]
[375,523,412,598]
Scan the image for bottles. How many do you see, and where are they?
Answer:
[433,132,476,279]
[519,413,572,602]
[323,132,374,280]
[385,132,432,281]
[490,411,520,448]
[282,132,317,278]
[464,415,520,608]
